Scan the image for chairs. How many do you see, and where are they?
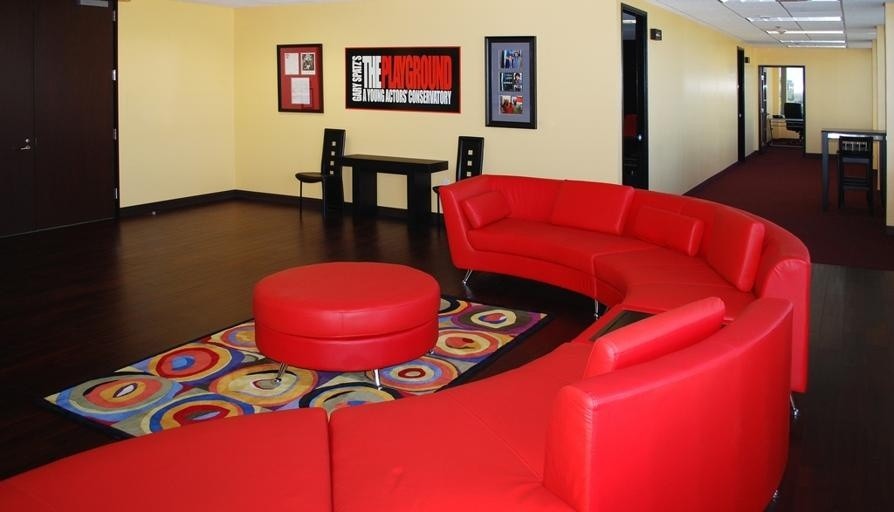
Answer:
[784,103,802,119]
[771,114,800,145]
[296,128,345,221]
[432,136,484,231]
[834,136,879,212]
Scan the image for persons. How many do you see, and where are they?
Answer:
[514,73,522,84]
[505,98,522,113]
[505,56,514,68]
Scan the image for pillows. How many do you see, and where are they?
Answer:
[584,295,727,375]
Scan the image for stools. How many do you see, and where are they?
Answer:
[252,260,442,388]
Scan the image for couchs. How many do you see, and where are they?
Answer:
[2,172,815,512]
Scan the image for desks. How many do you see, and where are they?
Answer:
[821,130,887,210]
[336,152,448,234]
[785,118,804,129]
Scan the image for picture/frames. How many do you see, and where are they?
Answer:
[277,43,324,113]
[345,47,460,112]
[484,36,537,129]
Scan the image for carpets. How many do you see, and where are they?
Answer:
[36,294,558,438]
